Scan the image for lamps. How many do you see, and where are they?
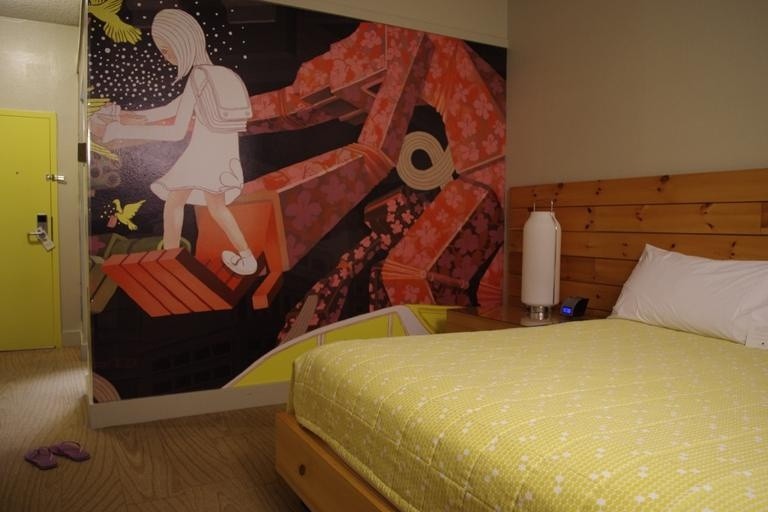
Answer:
[519,199,565,328]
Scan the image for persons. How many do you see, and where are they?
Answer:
[101,7,259,277]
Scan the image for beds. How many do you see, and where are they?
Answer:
[275,165,766,512]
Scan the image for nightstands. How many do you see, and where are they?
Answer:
[447,300,594,332]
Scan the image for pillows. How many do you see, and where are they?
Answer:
[604,242,767,353]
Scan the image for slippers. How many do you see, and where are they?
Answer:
[24,446,58,469]
[48,441,90,461]
